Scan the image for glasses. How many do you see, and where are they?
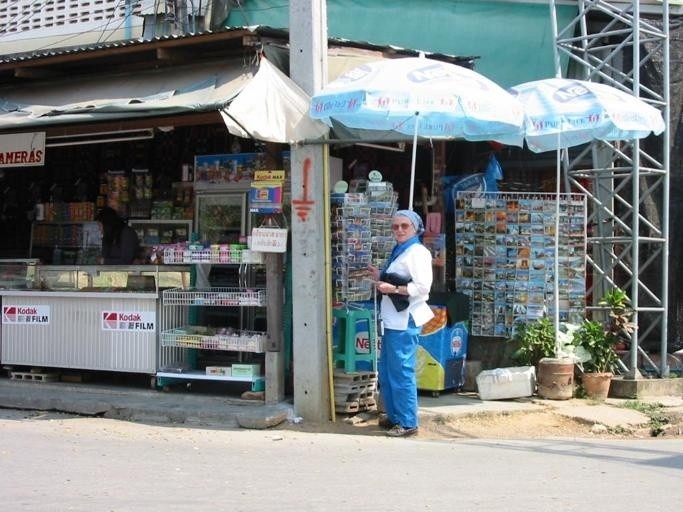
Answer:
[392,222,413,230]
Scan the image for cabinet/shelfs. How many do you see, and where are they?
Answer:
[127,219,192,265]
[33,190,104,263]
[195,194,245,363]
[0,260,190,384]
[249,194,267,363]
[157,244,265,389]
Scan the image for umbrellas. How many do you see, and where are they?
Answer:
[311,51,523,210]
[464,74,665,360]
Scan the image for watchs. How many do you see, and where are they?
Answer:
[395,285,399,295]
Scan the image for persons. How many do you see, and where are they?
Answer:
[366,209,433,438]
[95,207,143,265]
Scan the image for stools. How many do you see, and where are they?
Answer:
[332,308,378,373]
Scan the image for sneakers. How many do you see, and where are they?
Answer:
[378,417,418,437]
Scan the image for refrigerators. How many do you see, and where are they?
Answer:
[194,151,343,369]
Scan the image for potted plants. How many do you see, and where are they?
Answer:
[510,319,618,401]
